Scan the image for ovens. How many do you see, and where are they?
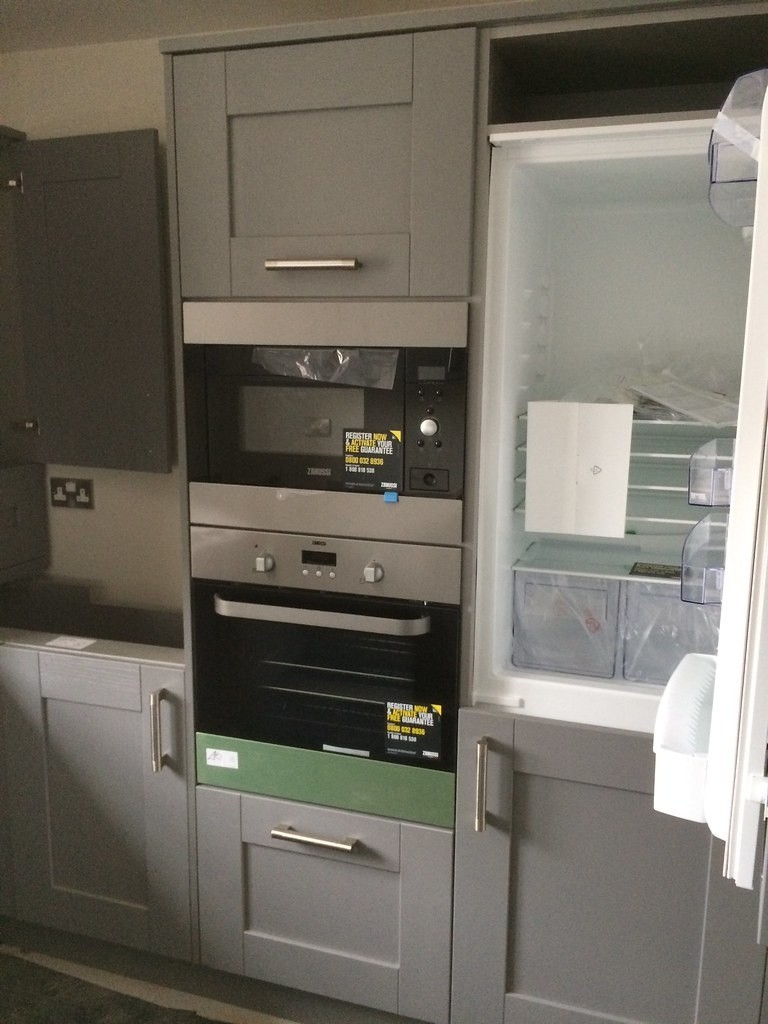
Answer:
[189,526,463,828]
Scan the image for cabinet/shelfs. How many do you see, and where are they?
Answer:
[448,702,766,1024]
[195,785,452,1024]
[162,24,481,297]
[0,643,195,998]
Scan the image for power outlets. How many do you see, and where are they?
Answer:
[49,476,95,509]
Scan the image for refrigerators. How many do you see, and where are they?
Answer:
[469,72,767,890]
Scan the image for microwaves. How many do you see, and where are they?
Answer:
[185,346,465,497]
